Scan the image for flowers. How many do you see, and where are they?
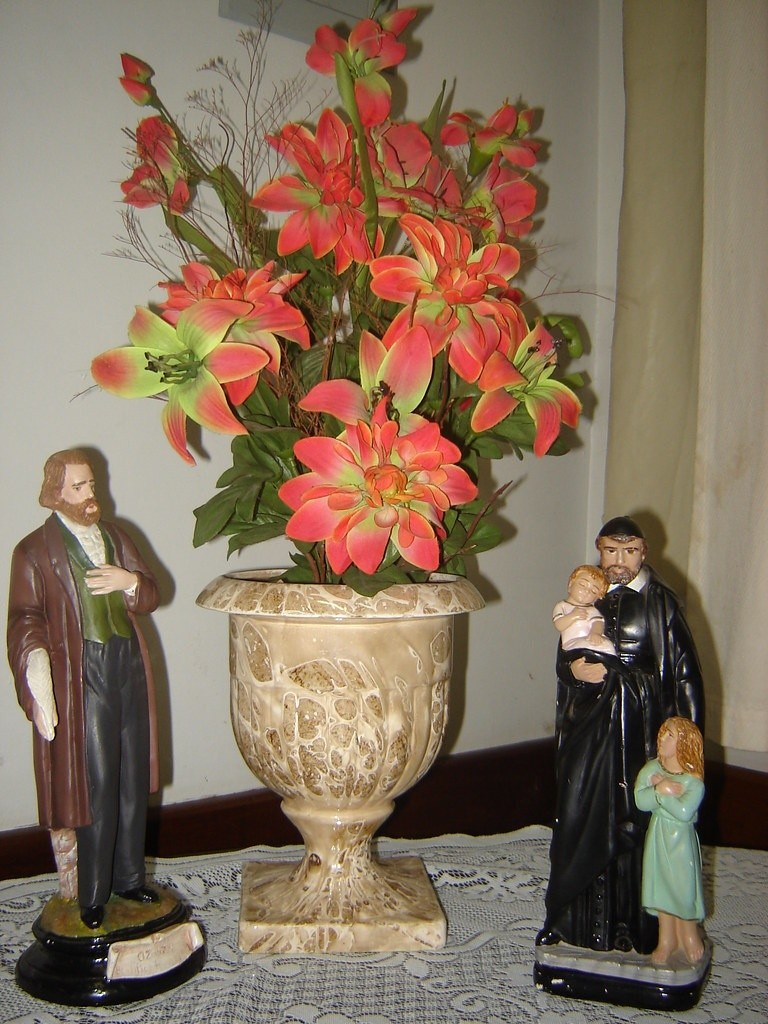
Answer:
[70,3,607,593]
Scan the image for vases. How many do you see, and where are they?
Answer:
[199,563,488,957]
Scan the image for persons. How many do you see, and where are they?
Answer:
[535,516,706,964]
[6,447,161,928]
